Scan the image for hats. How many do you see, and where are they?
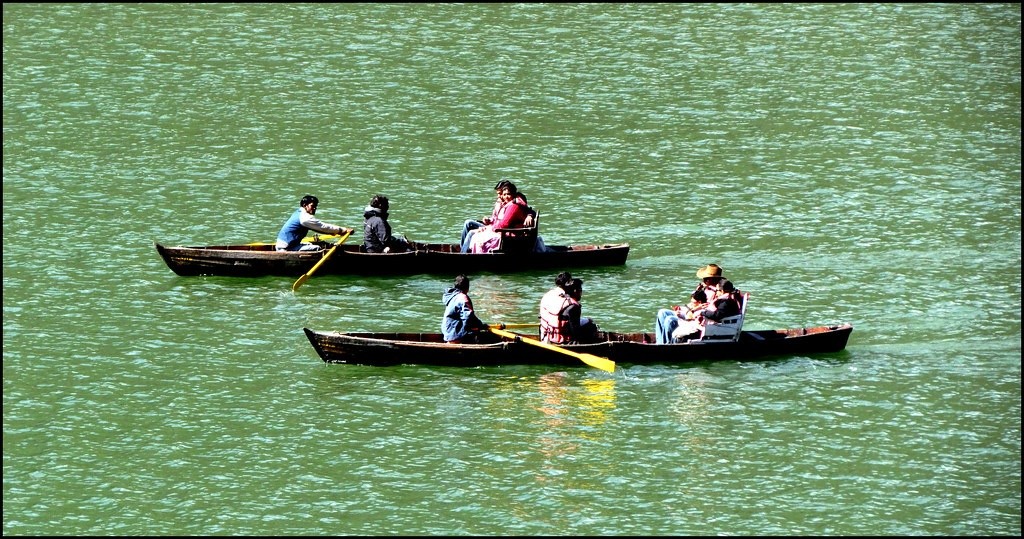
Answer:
[696,264,726,279]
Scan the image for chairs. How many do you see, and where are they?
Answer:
[490,210,540,253]
[686,293,749,344]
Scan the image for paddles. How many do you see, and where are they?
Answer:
[487,323,542,328]
[293,233,350,290]
[244,234,343,245]
[490,328,616,374]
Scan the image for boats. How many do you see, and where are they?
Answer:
[301,321,853,367]
[151,240,630,277]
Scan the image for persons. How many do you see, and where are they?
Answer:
[362,194,418,253]
[441,274,501,344]
[655,264,744,345]
[540,271,607,345]
[275,195,355,252]
[460,179,537,254]
